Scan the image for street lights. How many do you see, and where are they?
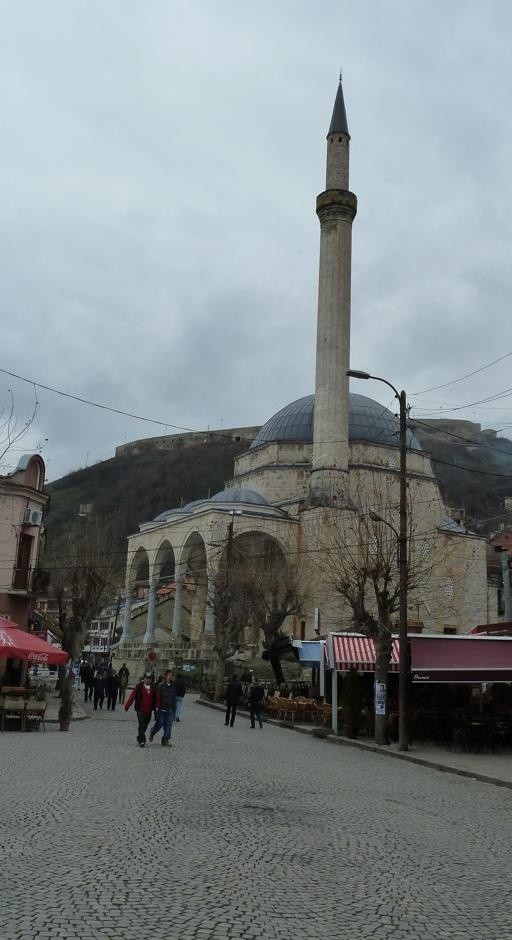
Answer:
[345,368,408,751]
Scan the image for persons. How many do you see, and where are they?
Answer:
[223,673,243,727]
[241,668,254,682]
[247,676,264,729]
[78,656,186,748]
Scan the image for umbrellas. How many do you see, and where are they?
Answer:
[0,614,71,728]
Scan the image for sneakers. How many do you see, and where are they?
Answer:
[135,728,176,747]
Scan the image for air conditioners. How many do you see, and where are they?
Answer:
[24,508,43,526]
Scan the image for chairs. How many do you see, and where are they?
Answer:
[267,695,512,754]
[0,685,48,732]
